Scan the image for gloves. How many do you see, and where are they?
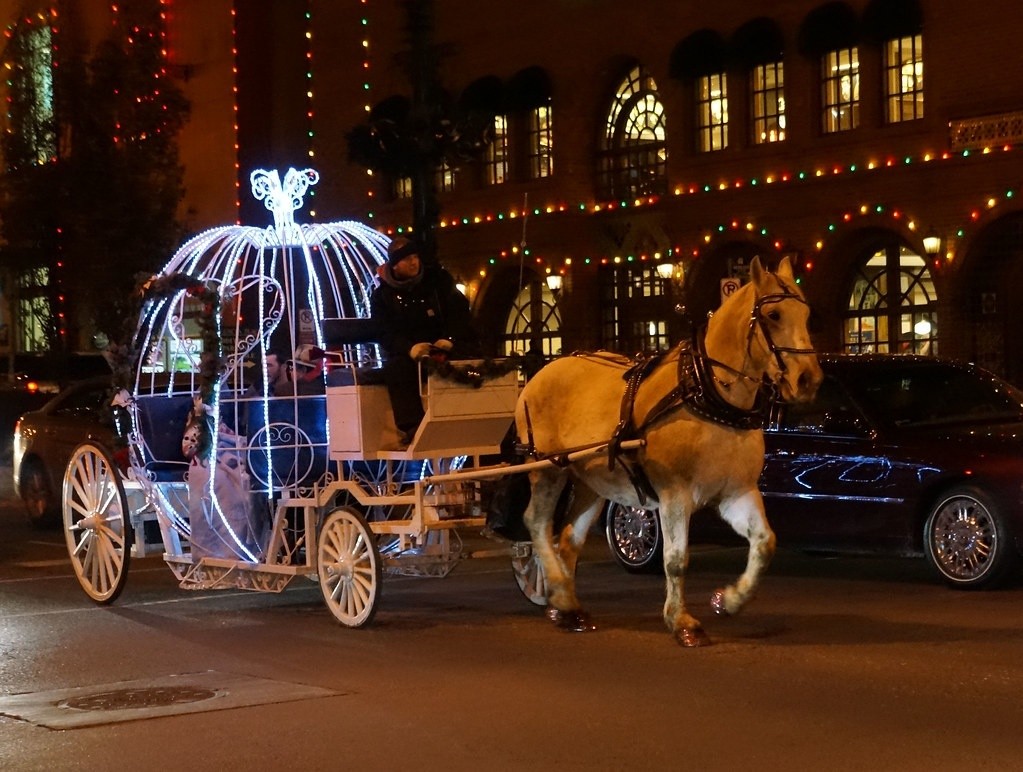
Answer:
[410,342,431,360]
[434,339,453,351]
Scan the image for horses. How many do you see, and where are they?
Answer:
[513,255,826,646]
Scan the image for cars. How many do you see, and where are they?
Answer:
[0,349,132,532]
[476,350,1023,591]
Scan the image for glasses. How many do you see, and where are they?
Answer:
[289,366,294,371]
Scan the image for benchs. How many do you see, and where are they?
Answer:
[137,394,244,481]
[246,397,430,493]
[321,318,387,386]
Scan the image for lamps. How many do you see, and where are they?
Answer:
[914,313,931,335]
[546,270,563,297]
[922,225,944,266]
[656,256,674,285]
[455,274,466,295]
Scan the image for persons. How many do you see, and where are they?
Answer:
[369,237,472,446]
[245,343,326,398]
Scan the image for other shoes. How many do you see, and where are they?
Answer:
[401,428,420,444]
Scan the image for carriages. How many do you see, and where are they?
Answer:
[58,166,827,649]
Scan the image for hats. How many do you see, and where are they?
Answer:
[287,344,324,369]
[388,239,416,264]
